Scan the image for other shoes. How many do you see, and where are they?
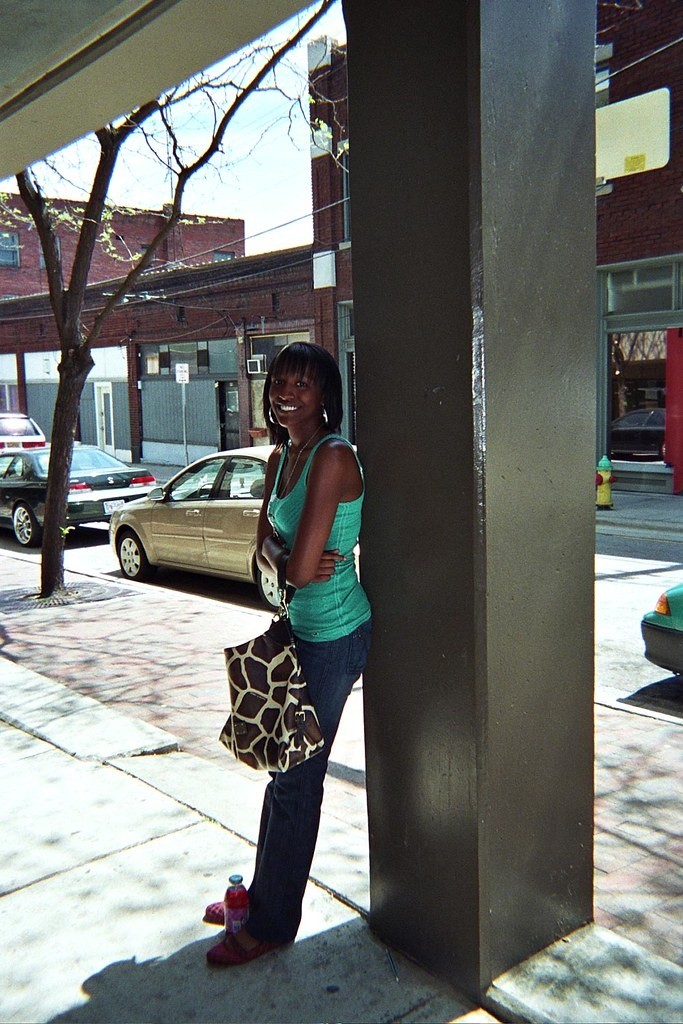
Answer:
[207,933,295,967]
[206,901,224,923]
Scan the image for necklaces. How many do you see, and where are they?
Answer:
[269,421,326,542]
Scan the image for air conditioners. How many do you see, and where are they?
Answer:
[247,359,264,374]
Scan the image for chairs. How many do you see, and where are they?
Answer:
[250,478,265,496]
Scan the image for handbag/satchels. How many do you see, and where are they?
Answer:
[217,551,326,773]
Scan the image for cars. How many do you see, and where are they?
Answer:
[108,445,284,613]
[0,413,47,451]
[0,444,159,548]
[639,582,683,675]
[608,408,665,461]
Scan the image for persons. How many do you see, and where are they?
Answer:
[203,342,371,967]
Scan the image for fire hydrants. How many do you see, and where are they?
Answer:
[597,453,617,509]
